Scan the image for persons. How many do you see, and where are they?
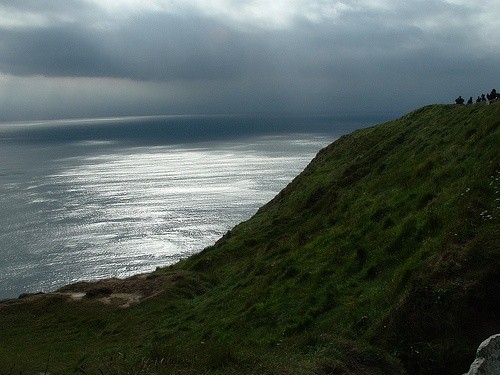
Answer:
[453,84,500,111]
